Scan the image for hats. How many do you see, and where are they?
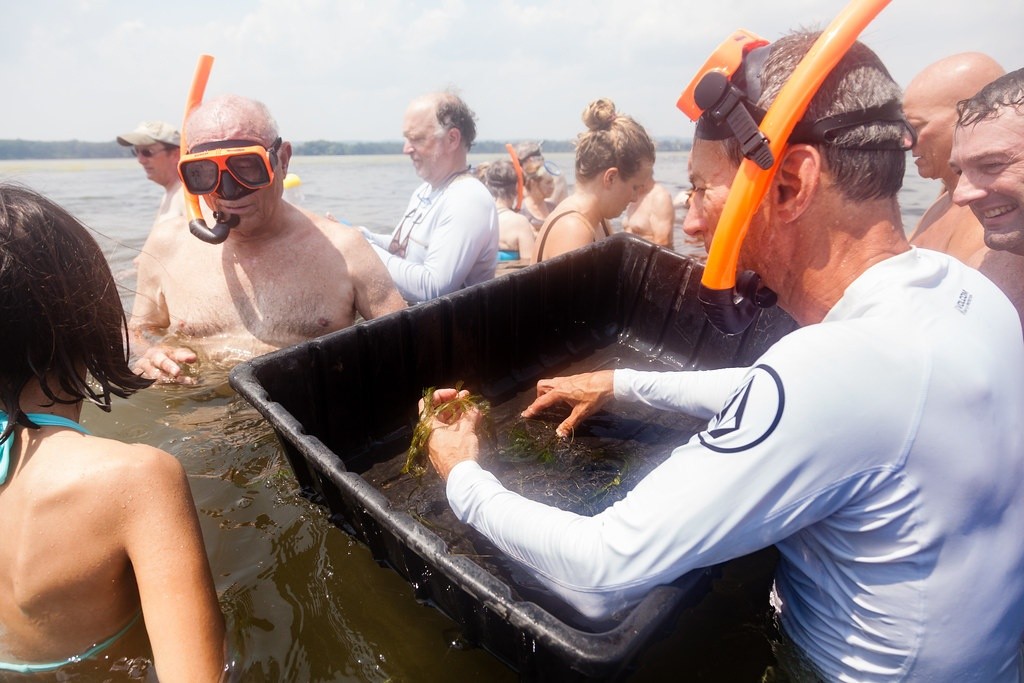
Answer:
[116,120,181,146]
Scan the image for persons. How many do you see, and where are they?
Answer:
[417,30,1024,683]
[901,52,1007,265]
[948,67,1024,332]
[0,185,228,683]
[115,91,674,385]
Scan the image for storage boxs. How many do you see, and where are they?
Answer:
[225,230,803,683]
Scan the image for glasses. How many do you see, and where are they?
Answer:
[130,147,171,157]
[530,160,559,183]
[675,28,775,170]
[177,134,282,196]
[389,209,422,258]
